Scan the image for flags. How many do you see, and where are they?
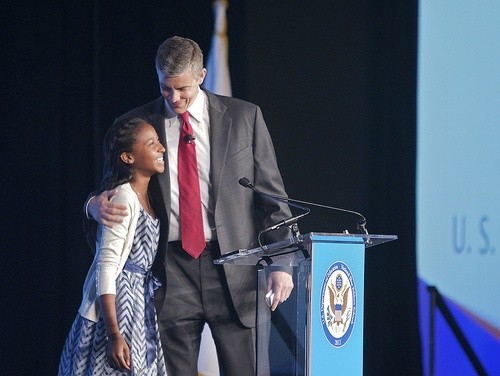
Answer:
[203,0,232,100]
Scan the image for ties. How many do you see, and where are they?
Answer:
[177,113,207,259]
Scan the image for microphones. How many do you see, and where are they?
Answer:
[238,177,367,234]
[185,134,192,143]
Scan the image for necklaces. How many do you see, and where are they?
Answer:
[131,181,153,218]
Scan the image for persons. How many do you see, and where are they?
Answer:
[56,116,165,376]
[81,36,299,376]
